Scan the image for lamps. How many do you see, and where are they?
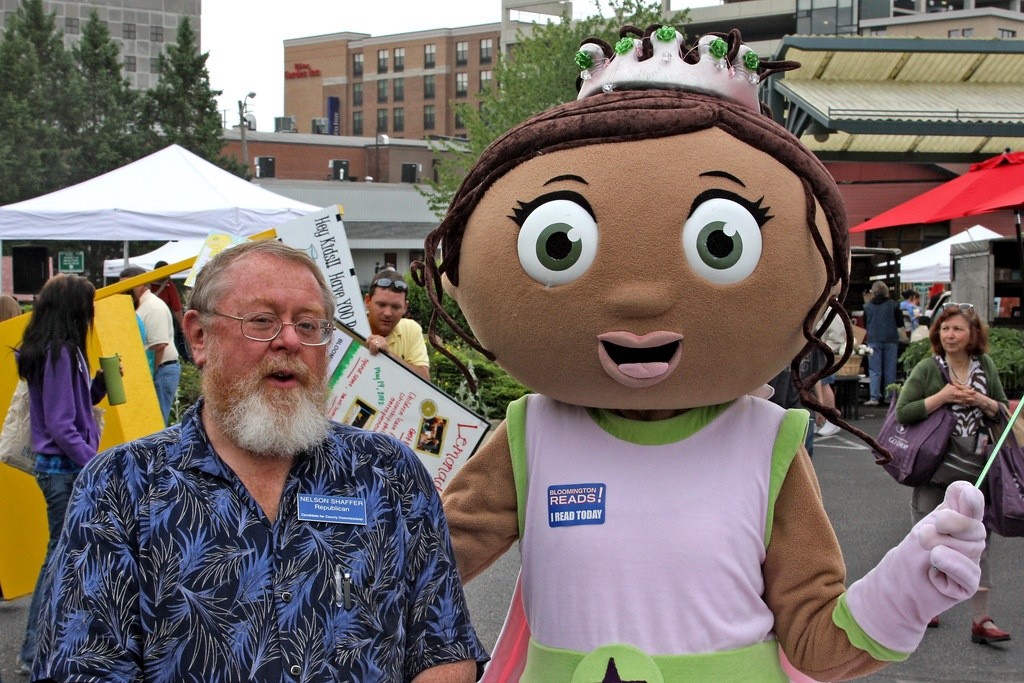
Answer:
[804,116,839,143]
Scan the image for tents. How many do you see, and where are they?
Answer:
[848,150,1024,234]
[0,143,326,271]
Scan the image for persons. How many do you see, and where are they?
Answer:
[6,274,124,542]
[863,281,921,405]
[896,302,1011,643]
[28,242,492,683]
[768,300,846,436]
[365,270,431,381]
[119,260,184,426]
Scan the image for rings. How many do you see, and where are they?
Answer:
[376,341,379,344]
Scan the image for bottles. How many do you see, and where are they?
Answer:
[98,354,126,406]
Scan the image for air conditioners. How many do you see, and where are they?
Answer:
[274,117,294,133]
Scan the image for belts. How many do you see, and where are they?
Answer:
[158,357,180,368]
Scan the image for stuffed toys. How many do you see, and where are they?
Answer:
[409,23,987,683]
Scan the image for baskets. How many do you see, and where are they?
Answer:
[833,354,863,376]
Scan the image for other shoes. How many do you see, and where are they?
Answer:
[813,418,841,436]
[14,655,33,674]
[883,395,892,402]
[863,399,879,405]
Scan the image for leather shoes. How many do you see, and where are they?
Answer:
[971,615,1011,643]
[927,616,939,627]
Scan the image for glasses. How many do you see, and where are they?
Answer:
[369,277,409,300]
[206,308,337,347]
[942,301,976,313]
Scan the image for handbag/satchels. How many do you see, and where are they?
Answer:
[984,403,1024,538]
[0,375,106,476]
[871,355,958,487]
[991,399,1024,450]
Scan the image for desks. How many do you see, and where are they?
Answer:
[833,376,861,420]
